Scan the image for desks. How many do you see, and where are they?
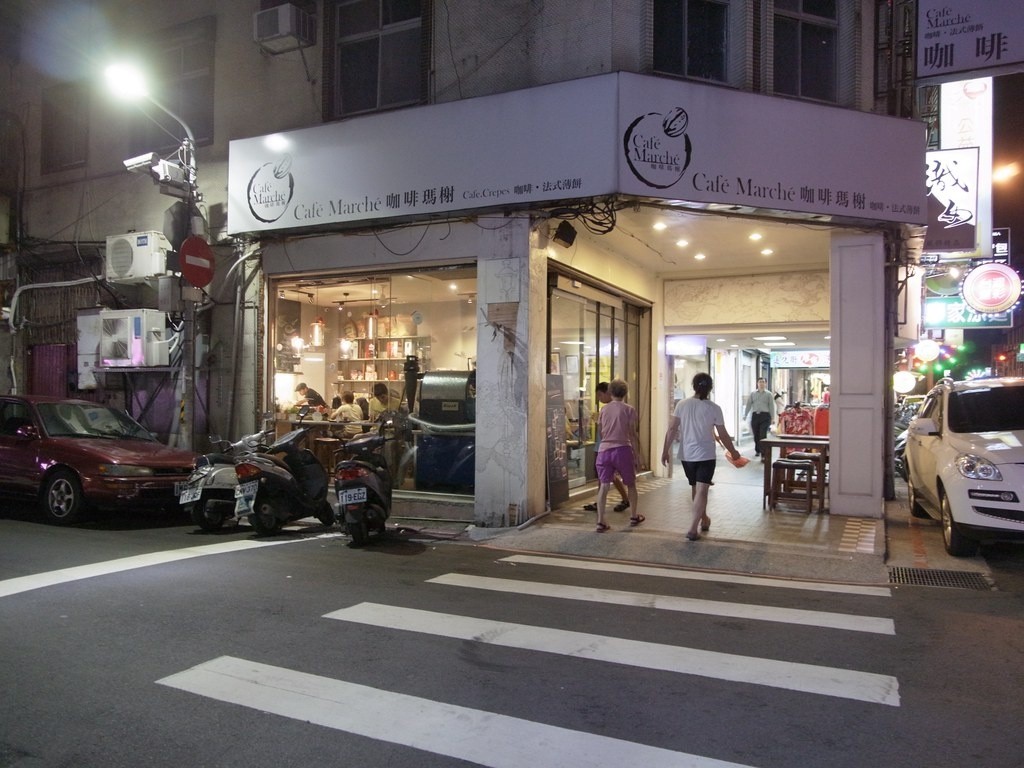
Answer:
[759,440,830,514]
[290,420,398,427]
[777,432,830,440]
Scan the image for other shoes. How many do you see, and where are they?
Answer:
[701,518,711,531]
[686,532,701,540]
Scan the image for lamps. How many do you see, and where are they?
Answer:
[892,371,916,393]
[365,277,378,341]
[310,284,325,348]
[553,220,578,250]
[891,333,941,363]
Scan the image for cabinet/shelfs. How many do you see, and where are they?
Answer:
[333,333,431,405]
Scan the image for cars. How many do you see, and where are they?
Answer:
[893,393,929,482]
[0,393,205,522]
[902,373,1024,557]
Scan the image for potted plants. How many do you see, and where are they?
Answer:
[288,407,299,422]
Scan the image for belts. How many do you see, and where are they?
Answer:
[753,412,770,415]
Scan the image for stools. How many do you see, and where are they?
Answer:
[770,451,822,516]
[314,437,352,477]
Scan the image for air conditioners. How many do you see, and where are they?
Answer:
[103,230,168,286]
[98,307,173,367]
[252,2,315,56]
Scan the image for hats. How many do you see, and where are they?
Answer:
[295,383,306,392]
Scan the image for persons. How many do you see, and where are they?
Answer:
[743,378,775,463]
[662,373,741,541]
[294,383,403,440]
[582,380,645,533]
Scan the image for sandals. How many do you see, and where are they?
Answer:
[597,523,610,532]
[583,503,598,510]
[614,501,629,512]
[630,514,645,526]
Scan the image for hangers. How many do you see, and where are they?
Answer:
[777,401,830,417]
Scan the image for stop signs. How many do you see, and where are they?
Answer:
[182,236,214,288]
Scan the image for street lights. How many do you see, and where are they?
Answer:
[108,68,203,450]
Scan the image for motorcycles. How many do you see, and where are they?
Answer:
[174,426,279,529]
[226,403,334,536]
[325,418,413,548]
